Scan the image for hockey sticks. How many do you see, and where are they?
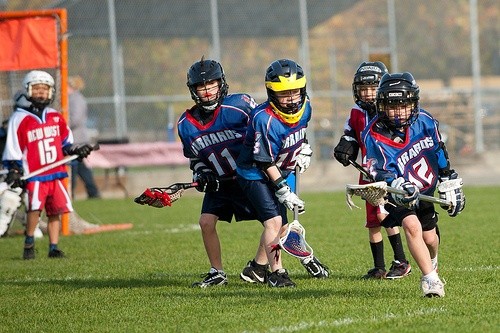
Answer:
[347,157,376,182]
[0,144,100,194]
[345,180,450,210]
[278,166,314,260]
[133,174,239,209]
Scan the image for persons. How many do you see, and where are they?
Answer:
[315,118,335,161]
[361,72,466,298]
[335,60,412,280]
[177,56,260,289]
[0,70,100,258]
[236,59,313,288]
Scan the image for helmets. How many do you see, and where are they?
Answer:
[186,60,229,113]
[375,72,420,129]
[352,61,388,111]
[265,58,307,123]
[24,70,55,105]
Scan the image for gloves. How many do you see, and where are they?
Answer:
[192,166,220,192]
[4,169,26,196]
[334,135,360,167]
[286,143,313,176]
[68,143,93,162]
[390,177,420,210]
[275,186,305,216]
[438,177,466,217]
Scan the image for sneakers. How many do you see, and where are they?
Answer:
[240,260,272,284]
[192,267,228,289]
[267,268,297,289]
[301,255,331,279]
[421,273,447,297]
[360,268,386,280]
[431,254,438,273]
[386,260,412,279]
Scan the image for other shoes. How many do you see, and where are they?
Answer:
[23,239,38,259]
[49,249,66,258]
